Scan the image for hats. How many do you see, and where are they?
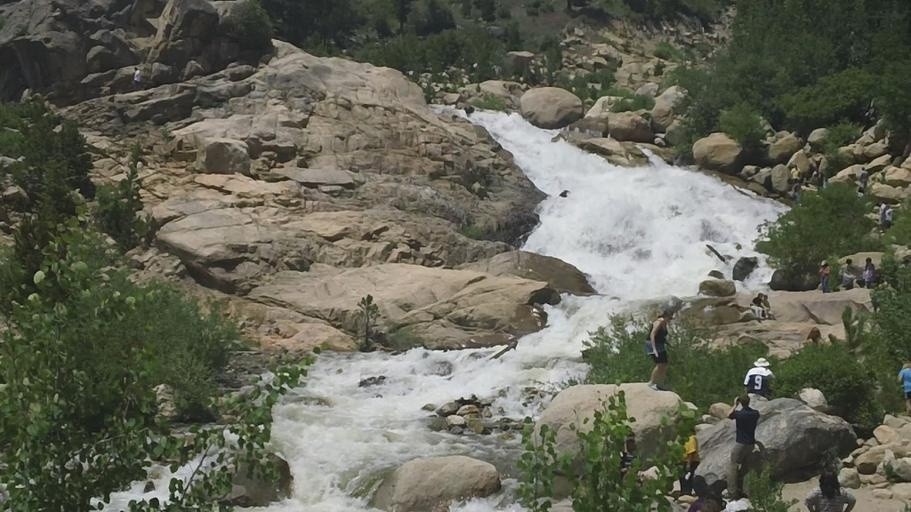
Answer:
[752,357,771,367]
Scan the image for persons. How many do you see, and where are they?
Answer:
[620,438,636,483]
[896,361,911,416]
[804,472,856,512]
[723,395,760,502]
[819,255,875,291]
[743,356,776,402]
[859,167,869,193]
[668,422,701,496]
[878,200,894,233]
[806,326,825,346]
[646,308,675,391]
[133,67,141,90]
[787,163,825,205]
[750,292,770,319]
